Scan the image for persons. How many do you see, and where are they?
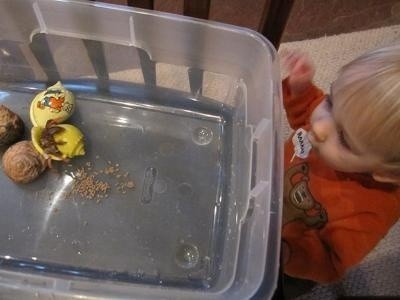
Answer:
[274,41,400,299]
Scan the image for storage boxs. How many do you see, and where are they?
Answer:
[0,0,284,299]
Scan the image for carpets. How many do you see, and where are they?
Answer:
[79,23,399,298]
